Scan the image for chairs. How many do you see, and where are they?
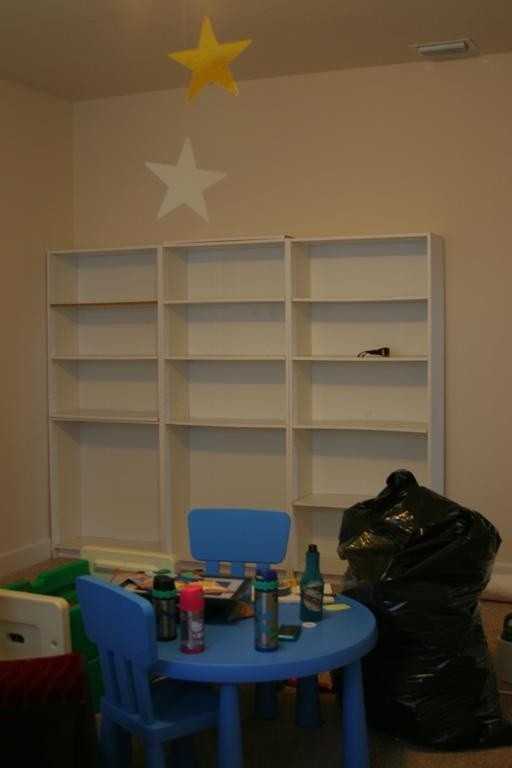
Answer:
[188,508,292,724]
[0,585,103,768]
[74,574,220,768]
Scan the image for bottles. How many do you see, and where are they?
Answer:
[300,544,324,622]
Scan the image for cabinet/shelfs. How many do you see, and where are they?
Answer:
[44,229,446,575]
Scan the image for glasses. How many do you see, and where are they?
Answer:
[356,348,389,357]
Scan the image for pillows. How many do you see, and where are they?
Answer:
[1,651,100,767]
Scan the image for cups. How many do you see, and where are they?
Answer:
[254,568,280,650]
[179,585,205,652]
[151,574,178,641]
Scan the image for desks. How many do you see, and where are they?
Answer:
[153,584,377,768]
